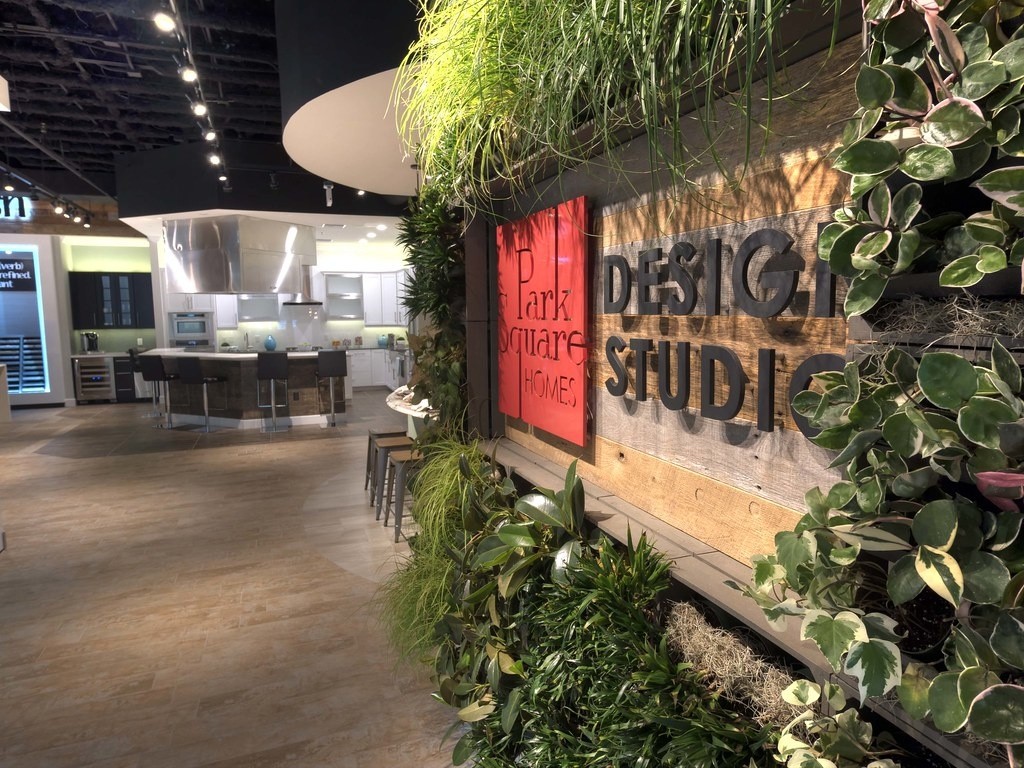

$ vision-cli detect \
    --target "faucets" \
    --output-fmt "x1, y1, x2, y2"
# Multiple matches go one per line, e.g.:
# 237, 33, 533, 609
243, 333, 254, 352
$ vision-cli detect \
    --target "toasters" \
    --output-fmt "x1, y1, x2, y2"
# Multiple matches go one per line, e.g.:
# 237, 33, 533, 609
81, 332, 105, 354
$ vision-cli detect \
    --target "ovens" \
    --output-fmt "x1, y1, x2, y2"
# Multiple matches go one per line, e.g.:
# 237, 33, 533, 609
72, 359, 115, 403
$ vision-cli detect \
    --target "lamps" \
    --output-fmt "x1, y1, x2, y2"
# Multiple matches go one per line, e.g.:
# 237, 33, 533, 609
153, 0, 231, 190
270, 172, 278, 189
4, 175, 16, 192
52, 195, 92, 229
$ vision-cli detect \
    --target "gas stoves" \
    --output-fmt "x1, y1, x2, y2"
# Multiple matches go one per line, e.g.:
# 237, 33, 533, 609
286, 346, 323, 351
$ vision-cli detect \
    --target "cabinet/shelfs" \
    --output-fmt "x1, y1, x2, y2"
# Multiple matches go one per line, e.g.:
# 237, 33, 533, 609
112, 356, 135, 402
347, 349, 387, 392
321, 270, 364, 320
132, 271, 155, 329
67, 271, 98, 331
214, 293, 238, 329
381, 268, 410, 327
168, 293, 214, 314
362, 272, 382, 327
385, 350, 400, 392
98, 272, 135, 330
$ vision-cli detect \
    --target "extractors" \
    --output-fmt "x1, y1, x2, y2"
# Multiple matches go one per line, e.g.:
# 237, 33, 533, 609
282, 293, 324, 305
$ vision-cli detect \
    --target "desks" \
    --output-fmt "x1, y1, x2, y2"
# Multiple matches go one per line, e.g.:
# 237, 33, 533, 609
386, 383, 440, 441
0, 364, 10, 416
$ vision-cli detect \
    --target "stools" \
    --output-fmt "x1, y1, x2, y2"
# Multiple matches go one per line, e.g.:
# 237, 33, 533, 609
315, 349, 348, 429
177, 357, 227, 433
139, 354, 187, 430
364, 427, 406, 501
370, 437, 416, 520
256, 351, 290, 433
384, 450, 425, 543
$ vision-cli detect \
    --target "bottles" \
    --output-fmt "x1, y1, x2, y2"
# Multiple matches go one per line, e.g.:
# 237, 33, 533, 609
378, 335, 387, 347
264, 336, 276, 350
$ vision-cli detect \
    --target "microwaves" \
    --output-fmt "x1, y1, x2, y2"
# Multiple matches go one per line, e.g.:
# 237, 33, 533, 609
168, 311, 217, 348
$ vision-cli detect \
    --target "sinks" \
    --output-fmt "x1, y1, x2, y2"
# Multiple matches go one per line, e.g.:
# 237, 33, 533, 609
228, 348, 257, 353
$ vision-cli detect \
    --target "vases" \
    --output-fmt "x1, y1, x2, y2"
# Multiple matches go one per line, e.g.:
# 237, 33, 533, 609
263, 336, 275, 351
